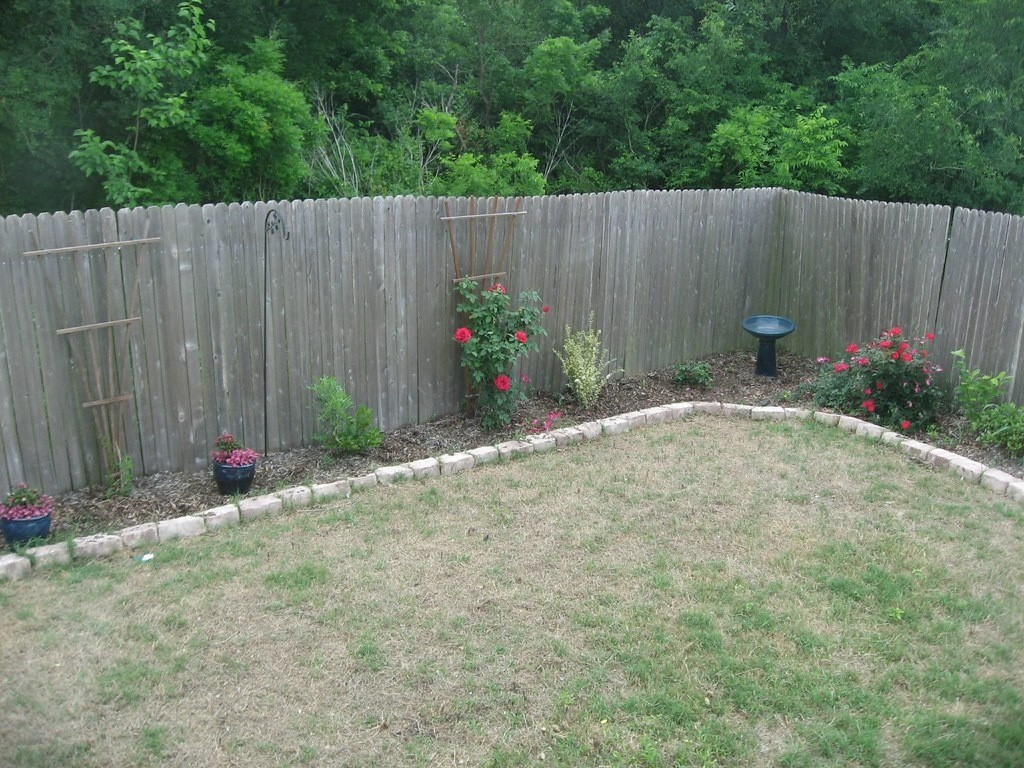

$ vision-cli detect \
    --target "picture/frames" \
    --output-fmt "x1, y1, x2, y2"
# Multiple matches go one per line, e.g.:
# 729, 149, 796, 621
212, 459, 255, 494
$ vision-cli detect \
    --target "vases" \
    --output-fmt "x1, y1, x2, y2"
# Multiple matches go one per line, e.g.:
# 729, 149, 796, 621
1, 512, 49, 542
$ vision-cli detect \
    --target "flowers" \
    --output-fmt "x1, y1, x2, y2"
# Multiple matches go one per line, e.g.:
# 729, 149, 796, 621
0, 480, 56, 520
211, 432, 258, 467
